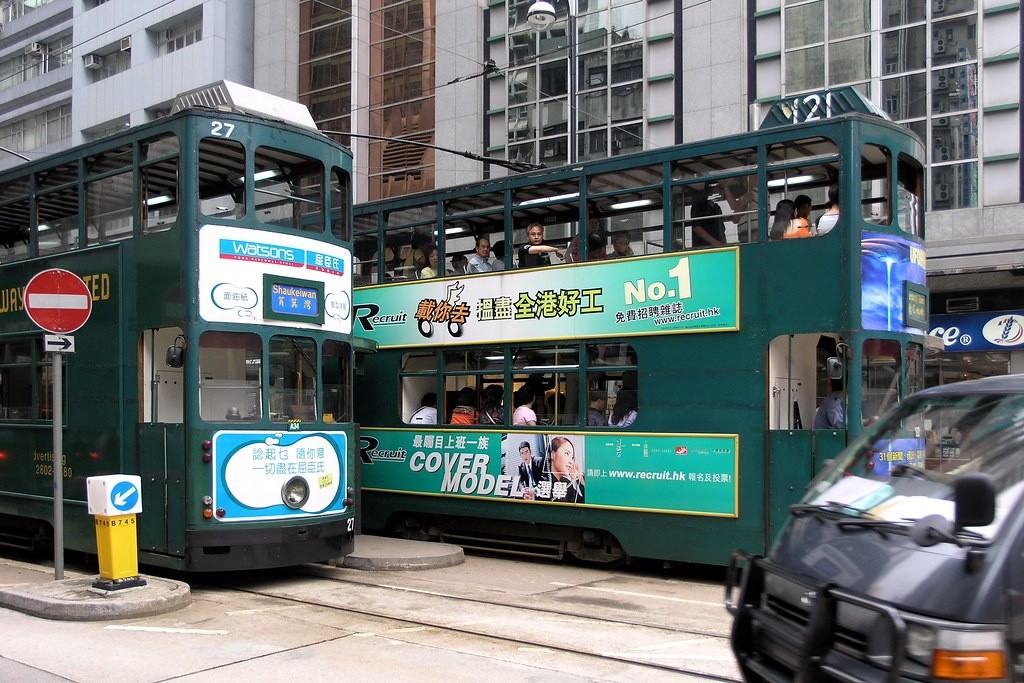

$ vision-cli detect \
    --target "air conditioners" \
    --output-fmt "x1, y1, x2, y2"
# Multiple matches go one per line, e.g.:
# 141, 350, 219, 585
934, 39, 946, 54
120, 37, 131, 54
84, 55, 104, 69
934, 110, 949, 127
933, 0, 945, 13
935, 75, 948, 91
935, 184, 950, 202
935, 147, 951, 163
24, 43, 47, 57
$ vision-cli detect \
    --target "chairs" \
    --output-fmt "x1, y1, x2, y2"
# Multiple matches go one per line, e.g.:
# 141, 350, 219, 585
393, 241, 575, 279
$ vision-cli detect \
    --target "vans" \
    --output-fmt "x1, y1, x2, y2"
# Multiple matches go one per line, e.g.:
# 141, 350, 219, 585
722, 372, 1024, 683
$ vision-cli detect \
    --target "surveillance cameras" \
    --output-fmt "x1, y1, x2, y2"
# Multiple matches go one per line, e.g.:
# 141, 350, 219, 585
527, 2, 557, 31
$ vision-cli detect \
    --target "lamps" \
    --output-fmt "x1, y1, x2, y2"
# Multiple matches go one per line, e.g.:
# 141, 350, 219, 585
28, 221, 63, 232
143, 190, 175, 206
227, 164, 292, 187
946, 297, 981, 313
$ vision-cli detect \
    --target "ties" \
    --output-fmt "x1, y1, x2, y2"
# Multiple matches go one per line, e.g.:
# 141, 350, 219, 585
528, 465, 535, 485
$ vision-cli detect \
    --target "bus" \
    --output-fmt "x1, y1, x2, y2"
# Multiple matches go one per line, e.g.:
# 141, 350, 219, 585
0, 79, 358, 573
264, 85, 945, 572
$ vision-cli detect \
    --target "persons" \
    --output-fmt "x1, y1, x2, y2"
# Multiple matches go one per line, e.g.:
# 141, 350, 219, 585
542, 436, 584, 502
812, 370, 875, 430
689, 179, 727, 248
401, 211, 634, 278
407, 347, 637, 426
517, 440, 545, 500
769, 179, 840, 240
718, 162, 772, 243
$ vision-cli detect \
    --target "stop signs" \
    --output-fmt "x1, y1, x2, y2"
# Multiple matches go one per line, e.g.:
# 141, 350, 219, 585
23, 267, 93, 336
